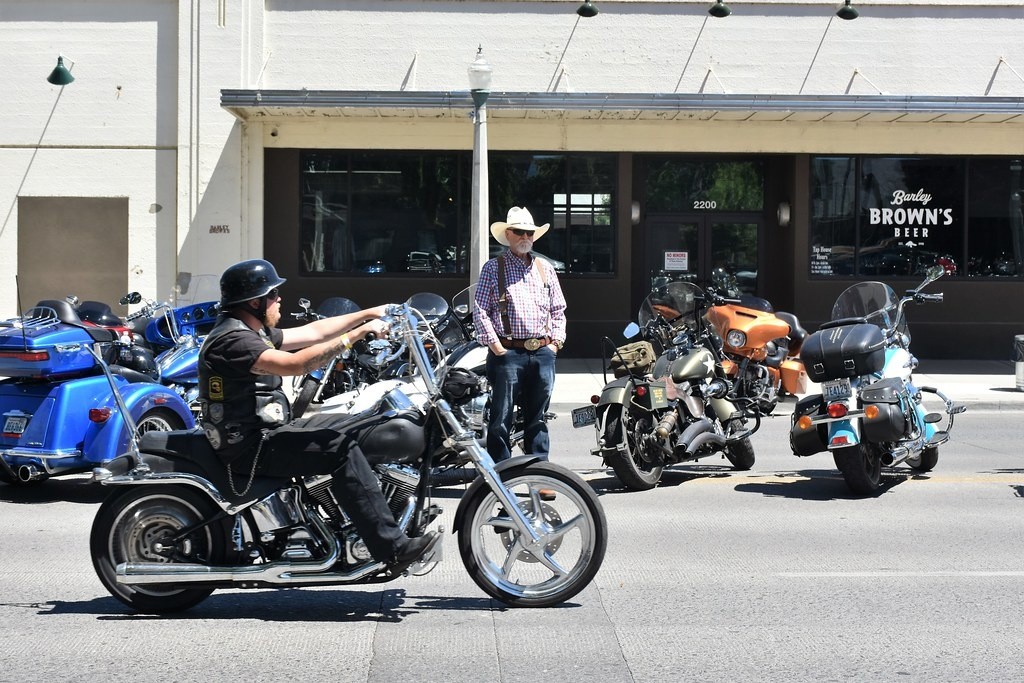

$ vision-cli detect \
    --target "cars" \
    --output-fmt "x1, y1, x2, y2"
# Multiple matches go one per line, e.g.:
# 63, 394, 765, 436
407, 244, 567, 275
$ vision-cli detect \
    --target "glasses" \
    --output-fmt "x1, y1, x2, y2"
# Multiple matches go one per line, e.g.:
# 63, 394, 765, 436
507, 228, 535, 237
267, 288, 278, 300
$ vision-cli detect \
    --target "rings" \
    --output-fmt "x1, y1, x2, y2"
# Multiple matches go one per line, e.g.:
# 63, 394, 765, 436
380, 326, 385, 331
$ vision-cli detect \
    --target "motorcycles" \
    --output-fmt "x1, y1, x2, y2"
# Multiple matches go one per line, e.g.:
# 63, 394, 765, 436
81, 298, 608, 616
650, 264, 809, 418
789, 260, 968, 496
0, 274, 557, 501
572, 278, 761, 492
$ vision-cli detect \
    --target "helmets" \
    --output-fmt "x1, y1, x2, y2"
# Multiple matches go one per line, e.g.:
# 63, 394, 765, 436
220, 260, 287, 307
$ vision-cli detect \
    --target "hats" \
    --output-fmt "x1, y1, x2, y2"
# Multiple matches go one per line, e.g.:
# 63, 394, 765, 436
490, 206, 550, 246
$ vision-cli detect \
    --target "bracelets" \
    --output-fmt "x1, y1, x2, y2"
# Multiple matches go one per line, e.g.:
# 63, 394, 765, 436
339, 333, 352, 350
551, 339, 563, 350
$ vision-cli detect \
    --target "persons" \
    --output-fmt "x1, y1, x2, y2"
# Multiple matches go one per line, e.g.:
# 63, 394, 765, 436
473, 207, 567, 501
198, 260, 439, 575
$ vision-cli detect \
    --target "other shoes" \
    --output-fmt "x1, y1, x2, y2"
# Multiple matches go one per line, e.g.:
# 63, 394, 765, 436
539, 489, 556, 500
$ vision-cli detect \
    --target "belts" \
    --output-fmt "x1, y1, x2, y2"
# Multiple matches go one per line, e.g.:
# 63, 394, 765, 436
499, 337, 550, 351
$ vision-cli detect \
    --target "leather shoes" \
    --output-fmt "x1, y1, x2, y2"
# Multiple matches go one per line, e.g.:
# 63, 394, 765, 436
406, 505, 438, 538
383, 531, 441, 577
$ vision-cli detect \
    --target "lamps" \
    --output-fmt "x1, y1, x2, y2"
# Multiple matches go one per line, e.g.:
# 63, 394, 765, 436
46, 54, 77, 87
839, 0, 858, 22
576, 1, 598, 17
707, 0, 730, 17
